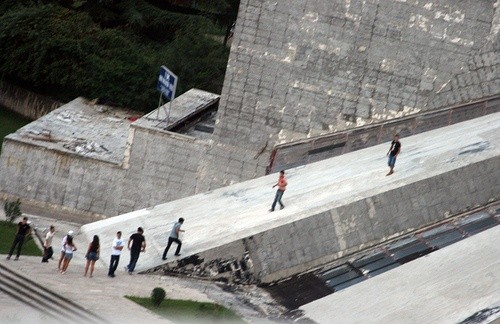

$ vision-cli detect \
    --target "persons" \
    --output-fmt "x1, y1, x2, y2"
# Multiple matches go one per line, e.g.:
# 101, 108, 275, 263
124, 226, 146, 275
386, 135, 401, 176
84, 235, 100, 278
57, 230, 77, 273
41, 227, 55, 263
162, 217, 185, 260
268, 170, 288, 212
108, 231, 124, 278
6, 217, 32, 261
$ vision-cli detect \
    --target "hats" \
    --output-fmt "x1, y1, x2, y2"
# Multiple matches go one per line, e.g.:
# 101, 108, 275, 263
67, 231, 74, 236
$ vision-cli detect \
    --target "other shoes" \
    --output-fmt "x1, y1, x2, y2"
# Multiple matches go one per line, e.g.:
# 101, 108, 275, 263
128, 271, 133, 275
173, 254, 180, 256
6, 256, 10, 260
269, 209, 274, 212
161, 258, 167, 260
14, 258, 18, 261
280, 207, 284, 210
62, 272, 65, 274
60, 270, 62, 273
57, 268, 60, 271
124, 266, 127, 271
